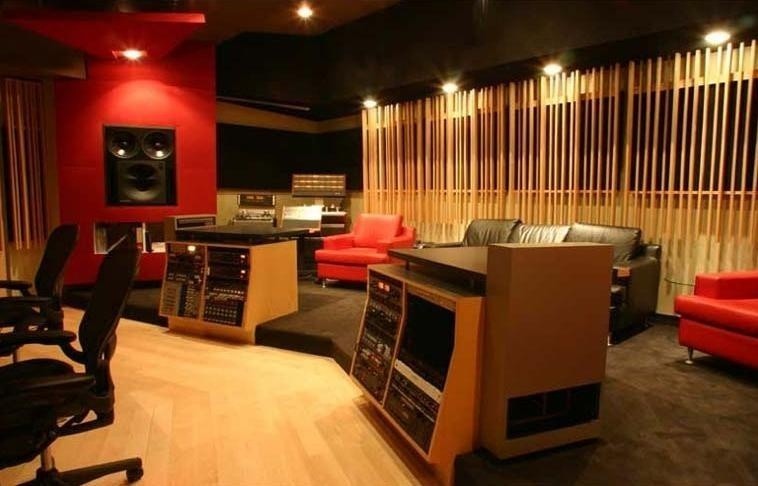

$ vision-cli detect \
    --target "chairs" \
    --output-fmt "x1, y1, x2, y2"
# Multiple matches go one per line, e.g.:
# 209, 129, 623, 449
0, 221, 86, 364
315, 211, 416, 288
0, 230, 147, 486
674, 270, 757, 374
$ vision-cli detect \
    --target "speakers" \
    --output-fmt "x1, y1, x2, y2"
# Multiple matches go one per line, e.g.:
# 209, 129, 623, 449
102, 123, 177, 207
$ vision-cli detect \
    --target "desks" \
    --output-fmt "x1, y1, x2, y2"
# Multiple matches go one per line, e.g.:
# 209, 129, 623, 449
185, 223, 311, 244
379, 233, 487, 289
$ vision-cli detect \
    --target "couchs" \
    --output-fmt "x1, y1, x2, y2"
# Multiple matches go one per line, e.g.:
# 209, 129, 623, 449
416, 214, 662, 347
